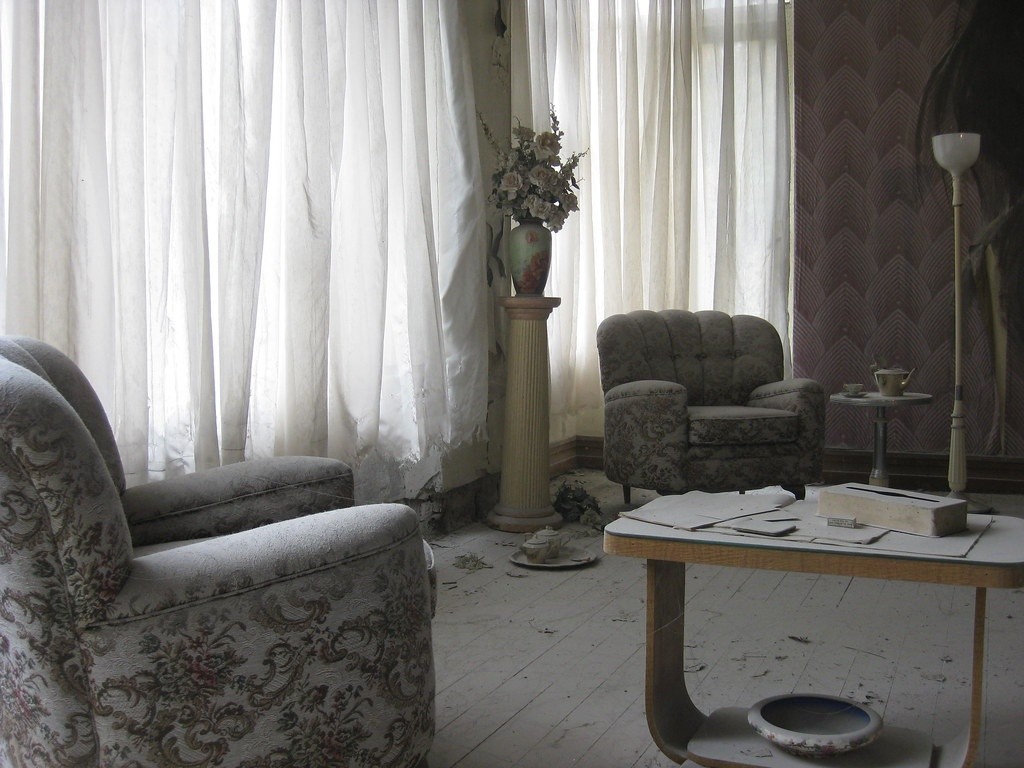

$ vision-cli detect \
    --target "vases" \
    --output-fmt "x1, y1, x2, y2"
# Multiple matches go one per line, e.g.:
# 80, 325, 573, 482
503, 214, 558, 299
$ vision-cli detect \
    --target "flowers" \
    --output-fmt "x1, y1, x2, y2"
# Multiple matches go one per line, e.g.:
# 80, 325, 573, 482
554, 478, 605, 534
482, 102, 589, 235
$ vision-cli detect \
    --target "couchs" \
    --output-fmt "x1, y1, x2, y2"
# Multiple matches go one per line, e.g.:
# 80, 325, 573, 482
594, 307, 826, 495
0, 334, 434, 768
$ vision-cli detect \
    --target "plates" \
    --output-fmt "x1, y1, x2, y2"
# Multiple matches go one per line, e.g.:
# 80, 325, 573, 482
839, 392, 868, 397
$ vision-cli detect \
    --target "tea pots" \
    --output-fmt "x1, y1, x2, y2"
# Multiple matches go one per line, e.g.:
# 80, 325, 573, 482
869, 364, 918, 397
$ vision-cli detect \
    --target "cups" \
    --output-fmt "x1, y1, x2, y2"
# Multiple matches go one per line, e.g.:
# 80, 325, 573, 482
843, 382, 864, 394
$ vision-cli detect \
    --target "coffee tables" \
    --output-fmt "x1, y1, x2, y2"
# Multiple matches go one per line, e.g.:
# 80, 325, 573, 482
830, 389, 933, 488
603, 490, 1024, 767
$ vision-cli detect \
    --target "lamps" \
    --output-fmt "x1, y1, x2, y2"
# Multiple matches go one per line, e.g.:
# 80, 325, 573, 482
930, 131, 994, 515
